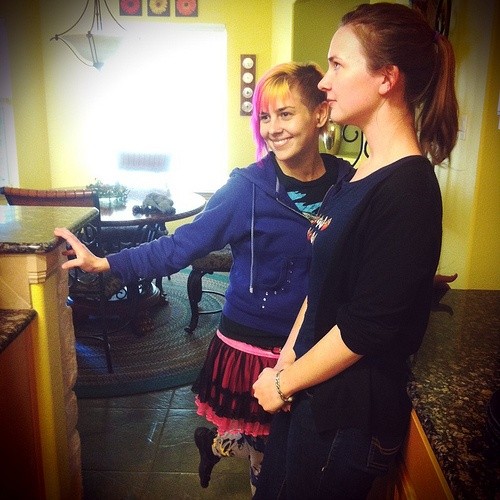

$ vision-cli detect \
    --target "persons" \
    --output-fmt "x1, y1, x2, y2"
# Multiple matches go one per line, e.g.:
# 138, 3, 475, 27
252, 2, 459, 500
54, 61, 357, 496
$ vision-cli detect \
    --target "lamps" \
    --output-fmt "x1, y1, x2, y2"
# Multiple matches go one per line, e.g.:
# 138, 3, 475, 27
49, 0, 126, 71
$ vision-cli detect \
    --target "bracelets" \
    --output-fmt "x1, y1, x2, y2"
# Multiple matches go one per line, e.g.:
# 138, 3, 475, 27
275, 369, 293, 402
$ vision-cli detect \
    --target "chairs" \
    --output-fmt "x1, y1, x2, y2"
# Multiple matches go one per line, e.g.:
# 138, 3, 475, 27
184, 243, 233, 332
0, 186, 146, 374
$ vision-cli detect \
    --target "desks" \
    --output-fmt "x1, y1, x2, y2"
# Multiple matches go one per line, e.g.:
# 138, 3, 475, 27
77, 191, 207, 337
